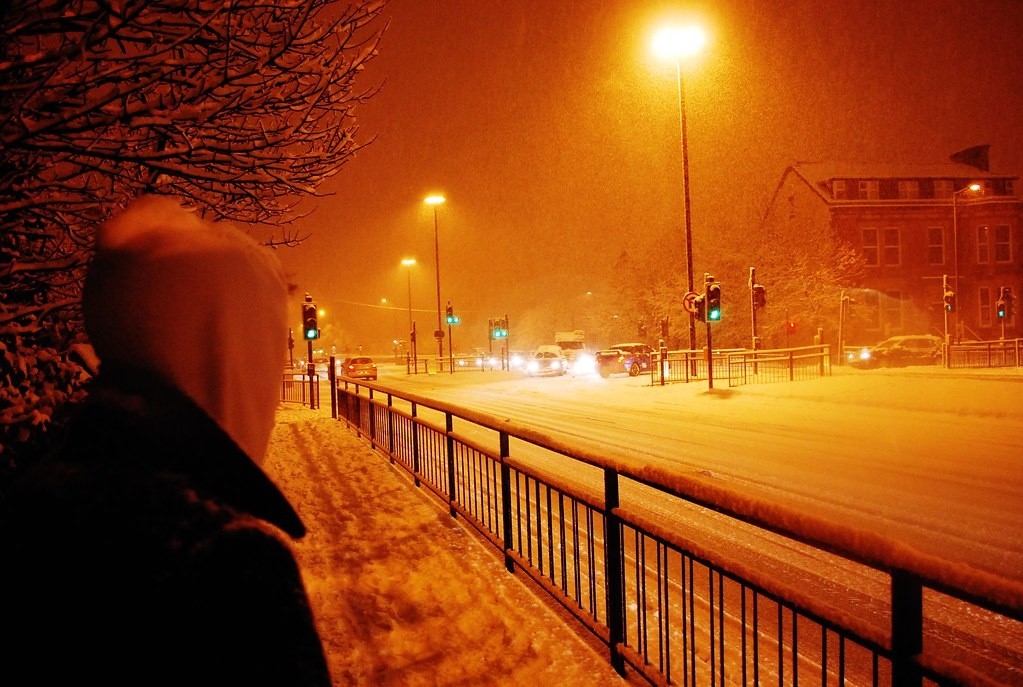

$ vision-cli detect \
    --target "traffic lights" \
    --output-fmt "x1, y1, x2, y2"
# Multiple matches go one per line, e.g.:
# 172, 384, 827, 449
494, 319, 507, 338
843, 295, 856, 327
996, 286, 1016, 320
302, 302, 318, 340
789, 321, 795, 333
753, 283, 766, 311
943, 289, 955, 312
446, 304, 460, 326
705, 282, 722, 322
288, 337, 294, 349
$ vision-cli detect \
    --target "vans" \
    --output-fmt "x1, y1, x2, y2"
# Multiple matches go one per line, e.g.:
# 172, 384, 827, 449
848, 334, 944, 370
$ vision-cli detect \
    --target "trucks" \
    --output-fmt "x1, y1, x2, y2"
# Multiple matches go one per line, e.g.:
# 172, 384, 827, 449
553, 331, 588, 372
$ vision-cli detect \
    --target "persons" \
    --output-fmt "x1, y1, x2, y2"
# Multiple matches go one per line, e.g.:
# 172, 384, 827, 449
0, 199, 332, 687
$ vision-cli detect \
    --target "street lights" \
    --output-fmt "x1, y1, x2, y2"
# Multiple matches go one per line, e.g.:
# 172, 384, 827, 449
318, 309, 325, 317
424, 195, 445, 371
400, 257, 414, 365
379, 297, 398, 362
654, 23, 698, 377
953, 183, 980, 340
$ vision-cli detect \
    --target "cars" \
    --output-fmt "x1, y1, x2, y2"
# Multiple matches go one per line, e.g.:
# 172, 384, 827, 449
340, 356, 377, 380
595, 343, 659, 378
505, 350, 526, 368
311, 358, 328, 372
469, 353, 496, 368
286, 359, 301, 368
453, 354, 469, 366
527, 346, 567, 377
